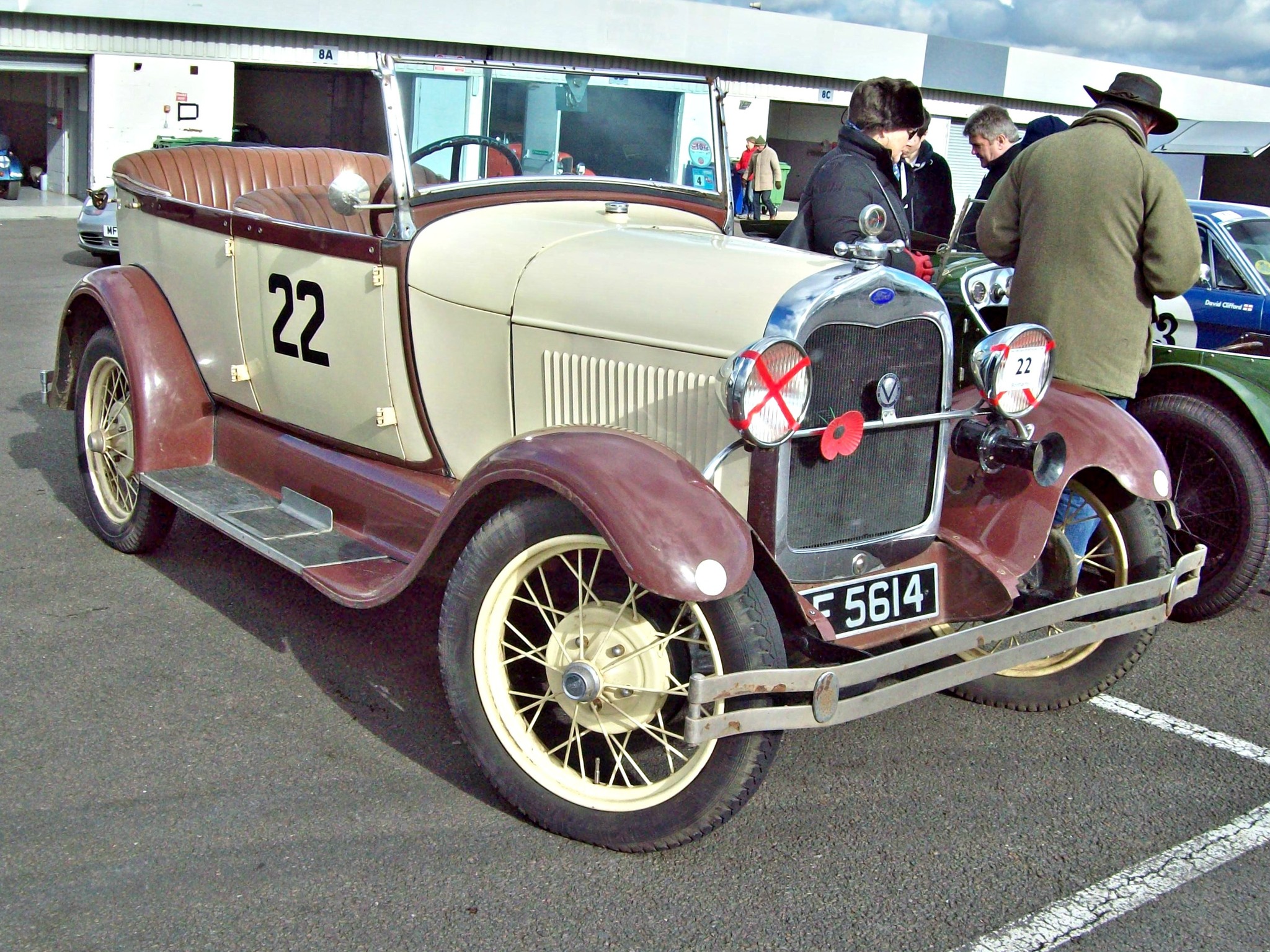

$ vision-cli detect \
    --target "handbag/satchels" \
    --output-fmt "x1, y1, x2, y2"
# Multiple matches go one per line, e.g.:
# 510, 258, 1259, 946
776, 199, 814, 250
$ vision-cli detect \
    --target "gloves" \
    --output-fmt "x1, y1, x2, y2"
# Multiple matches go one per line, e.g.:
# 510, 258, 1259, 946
904, 246, 935, 283
734, 164, 739, 170
741, 179, 746, 188
776, 181, 782, 189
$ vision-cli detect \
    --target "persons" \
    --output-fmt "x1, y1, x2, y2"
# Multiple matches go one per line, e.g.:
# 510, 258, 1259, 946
731, 136, 759, 221
741, 135, 784, 223
974, 68, 1205, 623
959, 104, 1020, 261
887, 106, 959, 257
785, 74, 939, 290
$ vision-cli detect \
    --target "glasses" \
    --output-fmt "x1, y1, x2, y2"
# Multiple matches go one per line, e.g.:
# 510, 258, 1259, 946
907, 130, 917, 138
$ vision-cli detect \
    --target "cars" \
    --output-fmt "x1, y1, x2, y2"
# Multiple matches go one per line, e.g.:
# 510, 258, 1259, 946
670, 197, 1270, 619
37, 35, 1205, 856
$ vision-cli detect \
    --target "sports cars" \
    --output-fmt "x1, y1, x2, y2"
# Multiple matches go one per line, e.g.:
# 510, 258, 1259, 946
75, 139, 281, 271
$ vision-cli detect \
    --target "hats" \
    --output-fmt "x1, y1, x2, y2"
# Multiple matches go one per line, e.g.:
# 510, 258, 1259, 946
746, 137, 757, 143
850, 76, 924, 129
755, 135, 766, 145
1084, 73, 1178, 135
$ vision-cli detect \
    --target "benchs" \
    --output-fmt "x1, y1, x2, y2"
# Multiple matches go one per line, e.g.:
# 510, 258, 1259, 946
112, 146, 441, 209
236, 185, 443, 243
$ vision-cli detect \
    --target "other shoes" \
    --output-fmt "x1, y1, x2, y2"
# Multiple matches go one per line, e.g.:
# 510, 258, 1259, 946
770, 207, 777, 220
737, 214, 754, 220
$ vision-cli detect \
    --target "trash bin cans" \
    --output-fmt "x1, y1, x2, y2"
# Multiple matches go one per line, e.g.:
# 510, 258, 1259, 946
728, 157, 791, 211
